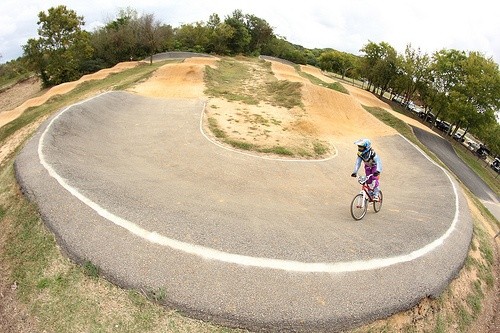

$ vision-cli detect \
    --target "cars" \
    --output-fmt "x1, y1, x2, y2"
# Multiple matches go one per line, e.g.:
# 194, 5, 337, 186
392, 94, 500, 173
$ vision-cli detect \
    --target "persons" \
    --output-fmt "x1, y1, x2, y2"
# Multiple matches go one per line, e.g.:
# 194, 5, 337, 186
351, 138, 382, 202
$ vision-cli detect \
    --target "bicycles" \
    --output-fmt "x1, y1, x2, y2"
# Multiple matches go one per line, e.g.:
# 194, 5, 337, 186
351, 173, 383, 220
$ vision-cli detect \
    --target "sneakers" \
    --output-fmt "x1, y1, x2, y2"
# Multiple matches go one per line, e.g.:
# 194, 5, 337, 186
372, 196, 379, 201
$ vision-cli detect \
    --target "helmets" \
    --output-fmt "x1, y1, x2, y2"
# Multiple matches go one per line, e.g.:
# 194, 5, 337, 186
354, 138, 371, 156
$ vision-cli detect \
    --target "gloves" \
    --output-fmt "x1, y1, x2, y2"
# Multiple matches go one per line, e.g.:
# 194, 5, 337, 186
373, 171, 379, 176
352, 172, 356, 177
